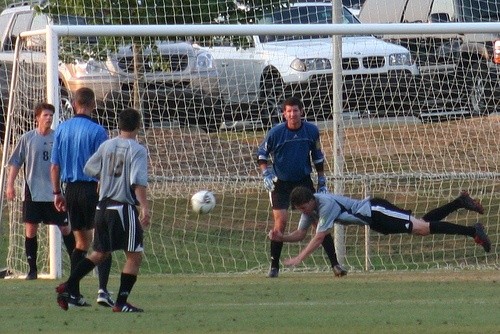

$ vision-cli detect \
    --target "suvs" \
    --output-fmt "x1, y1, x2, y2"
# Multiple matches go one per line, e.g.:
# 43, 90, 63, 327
0, 6, 219, 130
197, 7, 420, 124
357, 0, 499, 113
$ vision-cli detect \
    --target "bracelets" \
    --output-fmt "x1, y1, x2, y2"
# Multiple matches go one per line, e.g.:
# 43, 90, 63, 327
53, 189, 61, 195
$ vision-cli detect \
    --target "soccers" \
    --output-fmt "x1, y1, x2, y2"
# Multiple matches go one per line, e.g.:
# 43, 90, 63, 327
190, 190, 215, 214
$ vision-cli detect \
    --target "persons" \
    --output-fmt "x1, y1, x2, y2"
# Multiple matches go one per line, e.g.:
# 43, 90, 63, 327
54, 108, 149, 313
50, 88, 116, 306
257, 97, 347, 278
267, 186, 492, 267
5, 103, 79, 281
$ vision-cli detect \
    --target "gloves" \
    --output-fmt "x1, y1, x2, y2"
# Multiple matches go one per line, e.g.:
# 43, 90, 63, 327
317, 176, 328, 192
262, 170, 278, 192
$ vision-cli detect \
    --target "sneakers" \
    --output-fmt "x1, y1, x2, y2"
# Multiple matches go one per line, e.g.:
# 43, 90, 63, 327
472, 222, 491, 252
67, 292, 91, 307
269, 265, 280, 278
113, 301, 144, 312
55, 282, 69, 310
96, 289, 114, 307
26, 270, 38, 280
460, 190, 483, 215
332, 263, 347, 276
